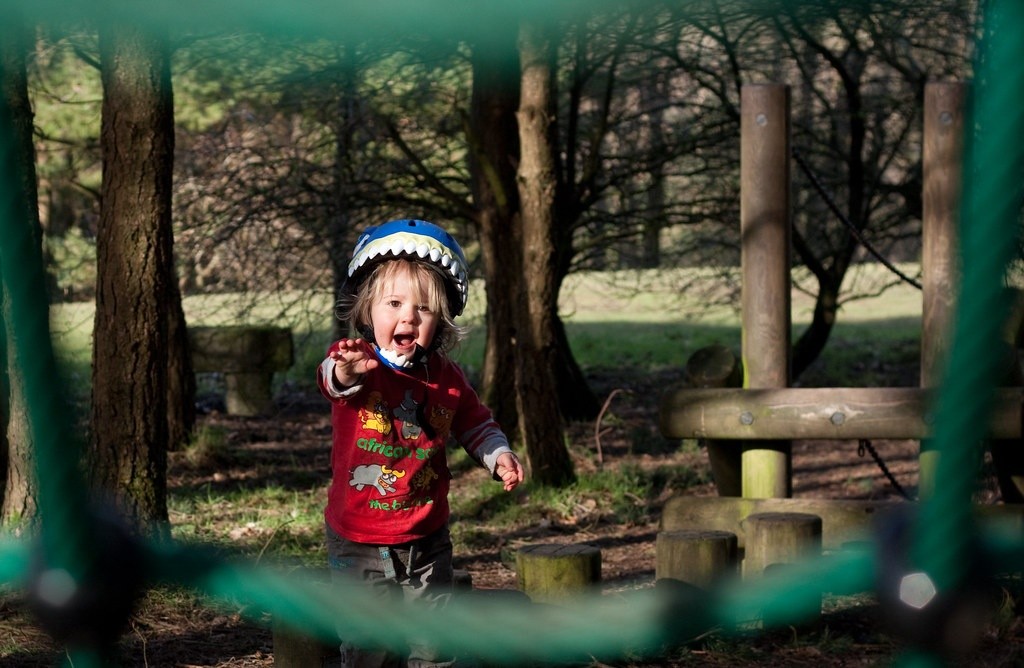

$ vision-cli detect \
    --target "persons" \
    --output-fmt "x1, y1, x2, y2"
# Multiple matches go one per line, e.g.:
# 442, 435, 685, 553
314, 216, 524, 668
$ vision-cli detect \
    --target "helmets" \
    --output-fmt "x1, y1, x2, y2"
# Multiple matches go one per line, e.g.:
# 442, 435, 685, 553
346, 218, 471, 373
